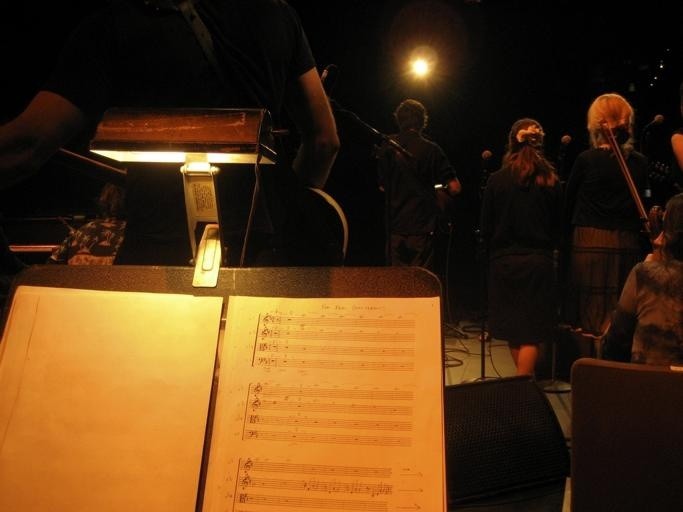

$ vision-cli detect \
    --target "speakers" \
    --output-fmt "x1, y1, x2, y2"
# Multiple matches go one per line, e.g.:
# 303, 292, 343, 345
445, 373, 570, 512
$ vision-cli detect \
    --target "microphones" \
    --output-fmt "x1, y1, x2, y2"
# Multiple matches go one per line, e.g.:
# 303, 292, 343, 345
557, 134, 573, 165
644, 113, 665, 134
482, 150, 493, 177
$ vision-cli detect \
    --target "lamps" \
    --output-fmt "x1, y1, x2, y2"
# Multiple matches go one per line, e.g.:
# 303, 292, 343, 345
89, 107, 276, 265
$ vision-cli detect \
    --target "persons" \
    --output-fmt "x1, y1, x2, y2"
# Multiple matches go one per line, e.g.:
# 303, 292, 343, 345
661, 82, 683, 195
45, 179, 132, 266
478, 116, 565, 380
563, 92, 650, 361
617, 189, 682, 368
374, 98, 463, 272
1, 0, 342, 266
